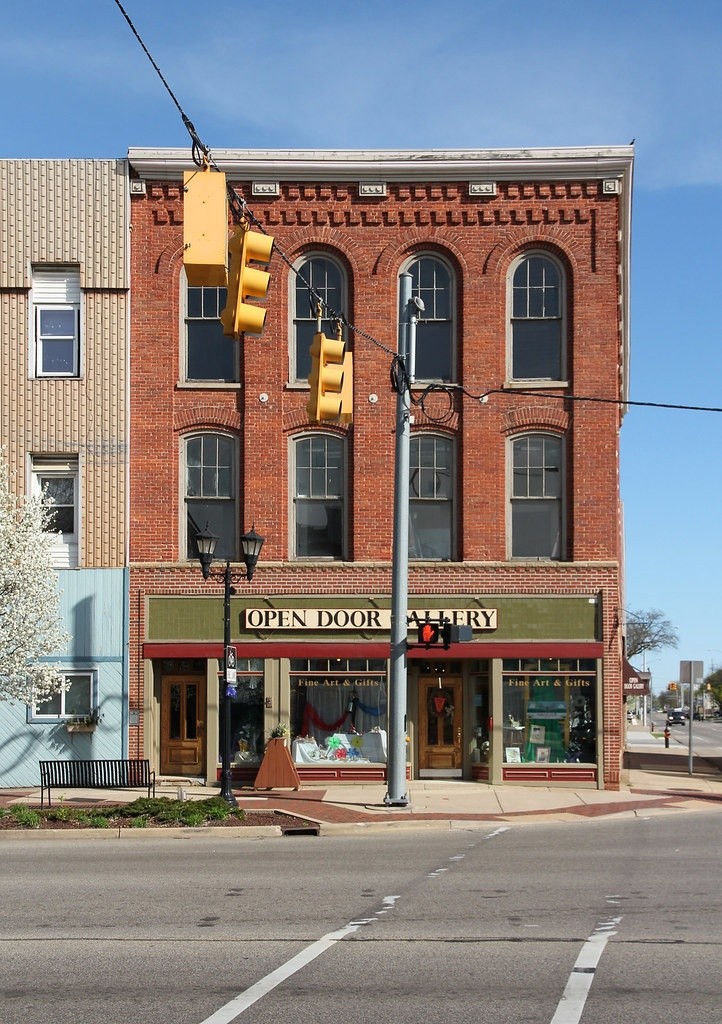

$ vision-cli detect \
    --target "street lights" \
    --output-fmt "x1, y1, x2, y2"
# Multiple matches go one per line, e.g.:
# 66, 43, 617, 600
197, 520, 266, 810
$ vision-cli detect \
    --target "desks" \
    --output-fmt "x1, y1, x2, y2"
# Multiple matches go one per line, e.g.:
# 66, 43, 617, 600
504, 727, 526, 760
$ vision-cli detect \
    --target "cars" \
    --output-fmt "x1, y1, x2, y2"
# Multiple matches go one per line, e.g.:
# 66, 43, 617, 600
667, 712, 685, 726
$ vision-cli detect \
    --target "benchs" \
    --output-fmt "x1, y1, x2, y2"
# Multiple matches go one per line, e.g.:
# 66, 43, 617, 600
39, 760, 156, 814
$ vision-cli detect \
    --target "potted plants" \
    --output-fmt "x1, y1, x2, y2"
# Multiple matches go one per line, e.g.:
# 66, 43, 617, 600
271, 725, 288, 747
58, 707, 104, 733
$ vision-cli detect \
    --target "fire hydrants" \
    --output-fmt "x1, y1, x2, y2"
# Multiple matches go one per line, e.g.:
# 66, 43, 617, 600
664, 726, 672, 748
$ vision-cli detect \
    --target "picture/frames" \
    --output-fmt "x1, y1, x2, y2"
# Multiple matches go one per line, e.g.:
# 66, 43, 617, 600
536, 747, 551, 763
530, 725, 545, 744
506, 748, 521, 763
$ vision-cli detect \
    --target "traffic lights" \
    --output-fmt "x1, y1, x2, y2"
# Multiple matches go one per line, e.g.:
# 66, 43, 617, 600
333, 321, 354, 427
418, 623, 439, 644
306, 301, 347, 426
669, 683, 676, 691
220, 213, 275, 339
